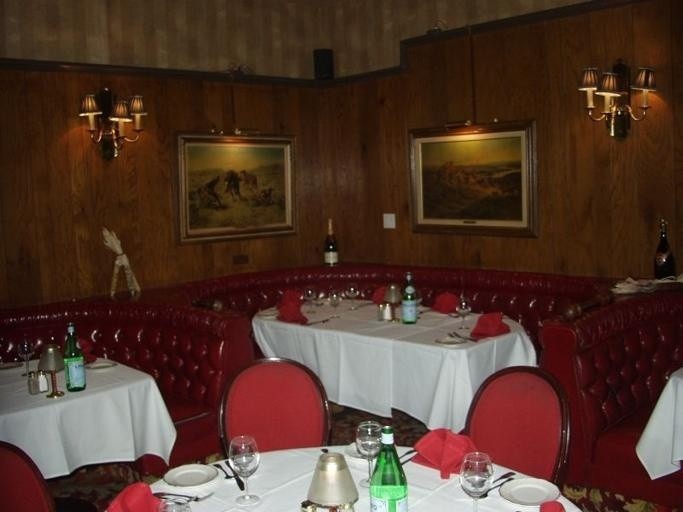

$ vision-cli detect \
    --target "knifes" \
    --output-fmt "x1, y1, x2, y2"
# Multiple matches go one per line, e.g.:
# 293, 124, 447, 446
223, 459, 246, 492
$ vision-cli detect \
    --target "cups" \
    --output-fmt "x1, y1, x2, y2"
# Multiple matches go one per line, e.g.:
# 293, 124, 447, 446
160, 499, 190, 510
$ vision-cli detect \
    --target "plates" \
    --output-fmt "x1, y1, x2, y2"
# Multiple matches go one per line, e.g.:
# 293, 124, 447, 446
85, 360, 117, 372
163, 463, 220, 488
434, 336, 466, 347
498, 476, 562, 508
346, 443, 377, 461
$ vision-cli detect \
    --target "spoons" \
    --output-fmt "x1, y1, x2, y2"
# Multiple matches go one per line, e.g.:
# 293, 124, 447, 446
478, 478, 513, 498
215, 464, 237, 480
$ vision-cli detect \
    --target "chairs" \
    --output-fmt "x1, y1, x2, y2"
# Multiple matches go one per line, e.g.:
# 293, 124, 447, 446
464, 367, 570, 485
217, 357, 332, 457
0, 440, 56, 512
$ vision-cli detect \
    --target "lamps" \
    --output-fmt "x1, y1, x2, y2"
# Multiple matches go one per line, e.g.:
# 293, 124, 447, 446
384, 283, 401, 323
579, 60, 656, 139
79, 87, 147, 157
37, 344, 65, 398
308, 452, 358, 512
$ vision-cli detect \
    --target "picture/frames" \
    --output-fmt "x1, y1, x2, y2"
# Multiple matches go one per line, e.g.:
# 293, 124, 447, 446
176, 129, 297, 243
407, 120, 538, 238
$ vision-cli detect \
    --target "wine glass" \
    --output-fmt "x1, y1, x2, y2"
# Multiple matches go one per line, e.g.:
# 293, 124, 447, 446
357, 420, 380, 490
259, 284, 360, 327
17, 342, 35, 376
460, 453, 494, 512
457, 296, 472, 330
229, 436, 262, 506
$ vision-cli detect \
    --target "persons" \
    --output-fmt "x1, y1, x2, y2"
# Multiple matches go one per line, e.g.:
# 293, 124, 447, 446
197, 168, 259, 209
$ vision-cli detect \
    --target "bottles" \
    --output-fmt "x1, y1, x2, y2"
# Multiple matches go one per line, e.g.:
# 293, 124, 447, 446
63, 323, 86, 392
369, 425, 409, 510
27, 371, 38, 394
377, 271, 417, 324
192, 299, 226, 312
36, 371, 50, 393
323, 218, 338, 267
654, 220, 675, 278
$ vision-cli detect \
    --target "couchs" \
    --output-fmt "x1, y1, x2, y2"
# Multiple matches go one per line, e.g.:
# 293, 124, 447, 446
538, 292, 682, 506
0, 296, 252, 478
166, 264, 624, 353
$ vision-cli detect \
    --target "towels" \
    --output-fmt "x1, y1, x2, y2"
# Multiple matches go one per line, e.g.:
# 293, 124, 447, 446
372, 288, 385, 303
430, 291, 459, 314
470, 311, 510, 338
105, 482, 166, 512
277, 288, 307, 323
411, 429, 476, 478
